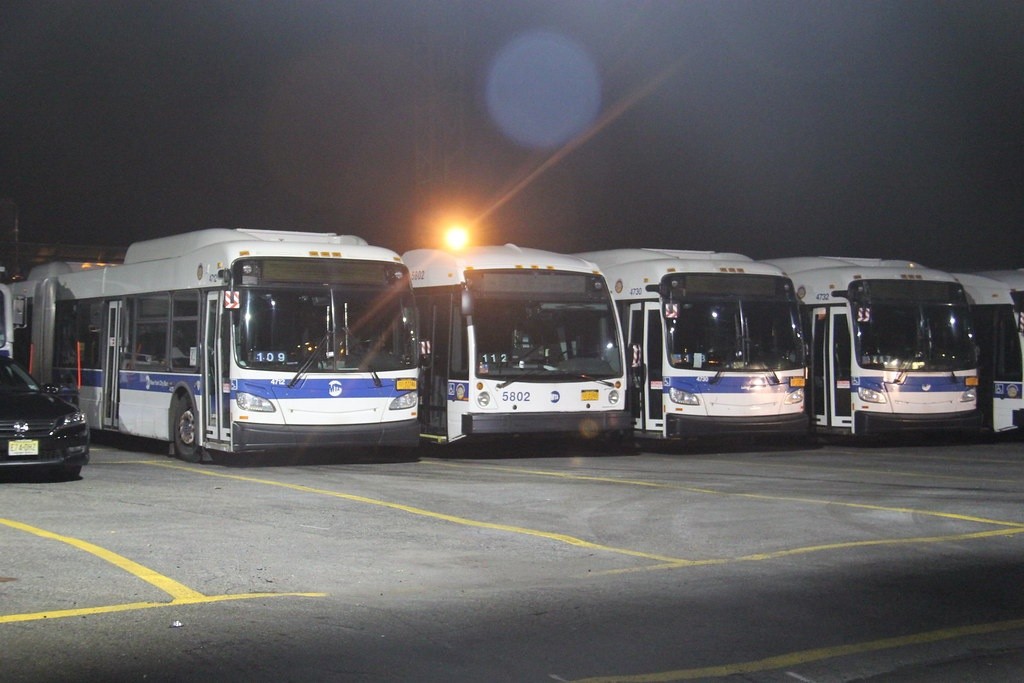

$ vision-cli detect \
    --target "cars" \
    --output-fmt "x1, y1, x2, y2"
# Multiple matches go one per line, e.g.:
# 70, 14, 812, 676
0, 354, 92, 484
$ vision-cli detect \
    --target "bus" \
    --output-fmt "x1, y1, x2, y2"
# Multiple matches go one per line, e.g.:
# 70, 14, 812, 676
945, 269, 1024, 439
566, 247, 812, 445
749, 256, 984, 441
399, 241, 642, 456
9, 226, 423, 463
